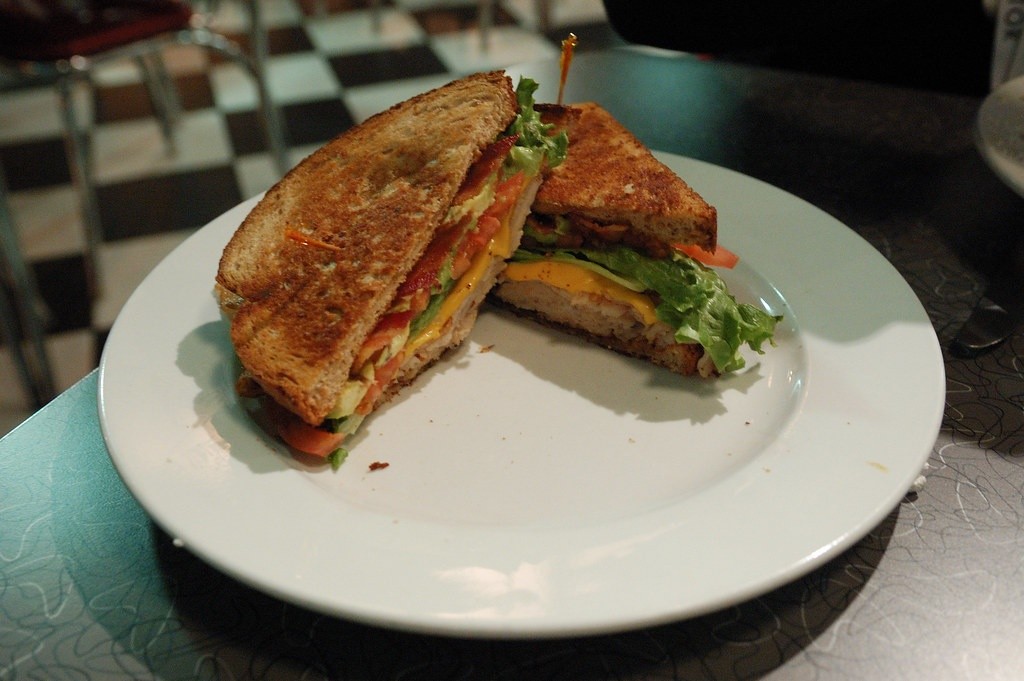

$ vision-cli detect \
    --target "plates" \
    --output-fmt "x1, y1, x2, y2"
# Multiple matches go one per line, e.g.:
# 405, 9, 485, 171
974, 77, 1024, 197
98, 150, 946, 638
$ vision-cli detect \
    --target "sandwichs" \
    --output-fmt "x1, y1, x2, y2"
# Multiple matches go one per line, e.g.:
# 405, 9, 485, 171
211, 68, 571, 459
486, 102, 783, 380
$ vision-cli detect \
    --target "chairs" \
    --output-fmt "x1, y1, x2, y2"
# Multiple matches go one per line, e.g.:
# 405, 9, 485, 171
0, 0, 289, 303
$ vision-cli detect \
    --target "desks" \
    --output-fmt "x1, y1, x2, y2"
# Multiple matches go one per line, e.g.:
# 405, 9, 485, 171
0, 48, 1023, 681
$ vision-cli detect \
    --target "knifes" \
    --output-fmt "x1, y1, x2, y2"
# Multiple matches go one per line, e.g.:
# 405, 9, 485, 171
954, 229, 1019, 347
